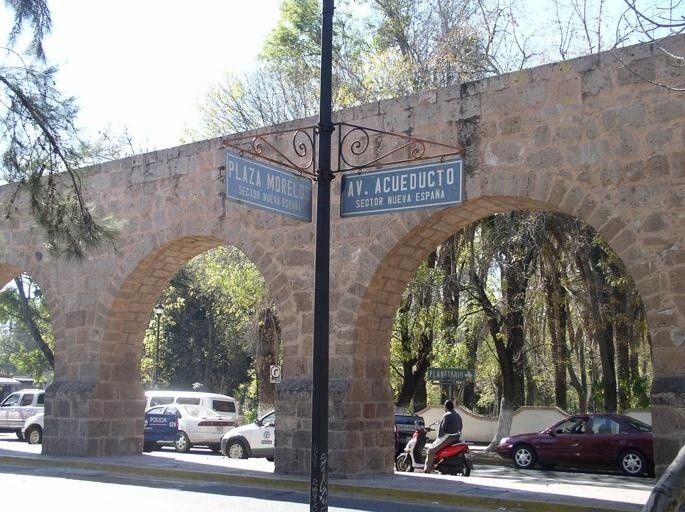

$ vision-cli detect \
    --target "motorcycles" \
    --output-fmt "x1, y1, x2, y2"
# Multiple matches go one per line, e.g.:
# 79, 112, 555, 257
397, 414, 475, 476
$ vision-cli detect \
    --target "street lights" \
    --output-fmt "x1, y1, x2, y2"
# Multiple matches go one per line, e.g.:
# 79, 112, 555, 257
151, 300, 166, 390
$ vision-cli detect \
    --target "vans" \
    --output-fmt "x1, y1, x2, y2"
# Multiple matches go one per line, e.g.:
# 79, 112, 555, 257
143, 391, 239, 430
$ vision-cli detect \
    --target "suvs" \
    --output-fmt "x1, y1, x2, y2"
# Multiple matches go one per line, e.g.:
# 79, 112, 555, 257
0, 388, 46, 439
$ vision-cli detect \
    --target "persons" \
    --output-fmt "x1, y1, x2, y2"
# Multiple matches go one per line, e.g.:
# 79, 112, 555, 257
420, 399, 463, 473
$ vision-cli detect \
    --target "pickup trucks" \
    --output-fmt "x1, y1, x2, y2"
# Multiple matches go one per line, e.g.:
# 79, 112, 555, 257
392, 411, 427, 463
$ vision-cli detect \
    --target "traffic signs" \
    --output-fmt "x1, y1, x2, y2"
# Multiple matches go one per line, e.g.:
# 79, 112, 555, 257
427, 366, 477, 383
335, 157, 466, 218
222, 151, 314, 224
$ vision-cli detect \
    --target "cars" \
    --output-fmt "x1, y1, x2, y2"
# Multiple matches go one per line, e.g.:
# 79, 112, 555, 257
221, 407, 276, 463
641, 442, 685, 512
492, 411, 656, 477
142, 404, 235, 454
21, 412, 45, 445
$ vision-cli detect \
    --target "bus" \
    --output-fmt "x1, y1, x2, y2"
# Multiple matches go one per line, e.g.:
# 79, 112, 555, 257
0, 376, 37, 412
